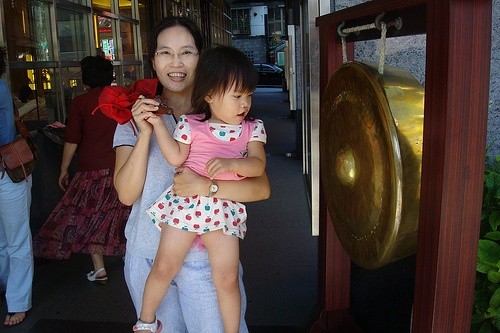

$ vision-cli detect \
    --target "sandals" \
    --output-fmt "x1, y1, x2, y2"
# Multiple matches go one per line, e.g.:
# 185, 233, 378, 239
87, 268, 108, 284
133, 312, 163, 333
3, 312, 26, 327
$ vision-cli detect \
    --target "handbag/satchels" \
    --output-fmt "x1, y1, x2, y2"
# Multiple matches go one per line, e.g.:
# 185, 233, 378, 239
0, 133, 40, 183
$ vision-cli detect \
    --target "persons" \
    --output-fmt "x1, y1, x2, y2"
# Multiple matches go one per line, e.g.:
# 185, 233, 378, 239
32, 55, 133, 282
132, 45, 267, 333
0, 44, 33, 327
113, 17, 271, 333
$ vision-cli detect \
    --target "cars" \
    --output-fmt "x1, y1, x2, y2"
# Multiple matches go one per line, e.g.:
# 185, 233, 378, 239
253, 63, 284, 86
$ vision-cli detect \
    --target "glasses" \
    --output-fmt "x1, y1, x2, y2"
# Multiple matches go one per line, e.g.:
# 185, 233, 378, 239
155, 49, 197, 59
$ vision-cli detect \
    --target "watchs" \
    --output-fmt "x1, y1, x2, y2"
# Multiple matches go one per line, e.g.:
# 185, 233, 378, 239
209, 181, 218, 197
